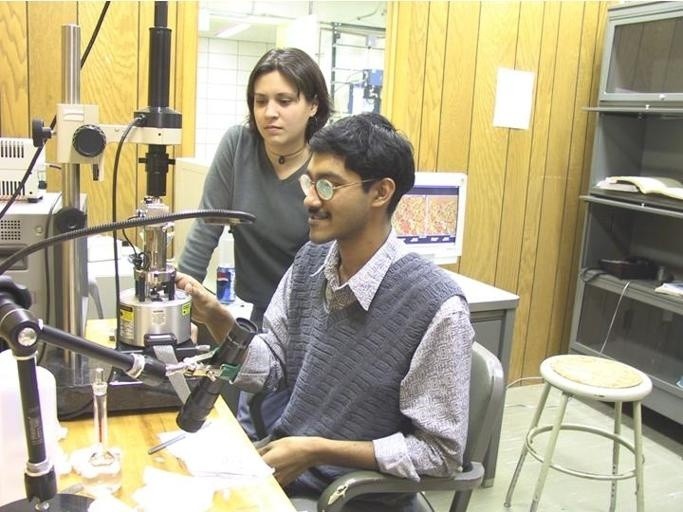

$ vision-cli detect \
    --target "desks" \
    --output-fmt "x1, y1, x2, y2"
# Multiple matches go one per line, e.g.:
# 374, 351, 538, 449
35, 312, 303, 510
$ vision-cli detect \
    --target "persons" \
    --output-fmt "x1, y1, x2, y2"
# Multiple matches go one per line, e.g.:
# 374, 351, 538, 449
176, 48, 337, 436
174, 113, 475, 510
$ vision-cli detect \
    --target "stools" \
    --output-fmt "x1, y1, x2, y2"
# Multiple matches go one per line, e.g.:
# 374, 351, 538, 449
499, 355, 653, 512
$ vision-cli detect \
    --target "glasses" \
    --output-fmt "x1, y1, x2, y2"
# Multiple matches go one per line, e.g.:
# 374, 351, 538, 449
296, 174, 378, 201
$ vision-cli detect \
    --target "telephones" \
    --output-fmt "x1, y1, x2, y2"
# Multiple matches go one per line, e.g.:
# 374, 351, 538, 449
599, 256, 658, 279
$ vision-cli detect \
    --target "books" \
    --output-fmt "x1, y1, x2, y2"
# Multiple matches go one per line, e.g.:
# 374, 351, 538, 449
596, 175, 683, 200
653, 281, 683, 299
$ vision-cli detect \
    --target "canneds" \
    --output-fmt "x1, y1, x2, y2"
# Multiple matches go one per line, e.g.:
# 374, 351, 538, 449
217, 267, 235, 305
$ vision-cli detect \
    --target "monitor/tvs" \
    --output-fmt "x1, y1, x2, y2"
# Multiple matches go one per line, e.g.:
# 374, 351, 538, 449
391, 172, 467, 264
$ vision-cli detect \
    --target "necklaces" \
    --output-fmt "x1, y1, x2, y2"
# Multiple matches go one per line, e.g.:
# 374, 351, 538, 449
268, 143, 307, 165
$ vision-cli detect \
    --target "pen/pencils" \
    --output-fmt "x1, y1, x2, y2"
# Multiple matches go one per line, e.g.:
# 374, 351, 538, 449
148, 434, 186, 454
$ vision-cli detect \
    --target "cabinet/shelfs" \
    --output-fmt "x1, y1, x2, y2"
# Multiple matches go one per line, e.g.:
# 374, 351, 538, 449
568, 1, 683, 428
438, 264, 517, 490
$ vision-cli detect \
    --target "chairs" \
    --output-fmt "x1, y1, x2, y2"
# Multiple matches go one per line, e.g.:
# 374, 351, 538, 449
306, 340, 503, 512
